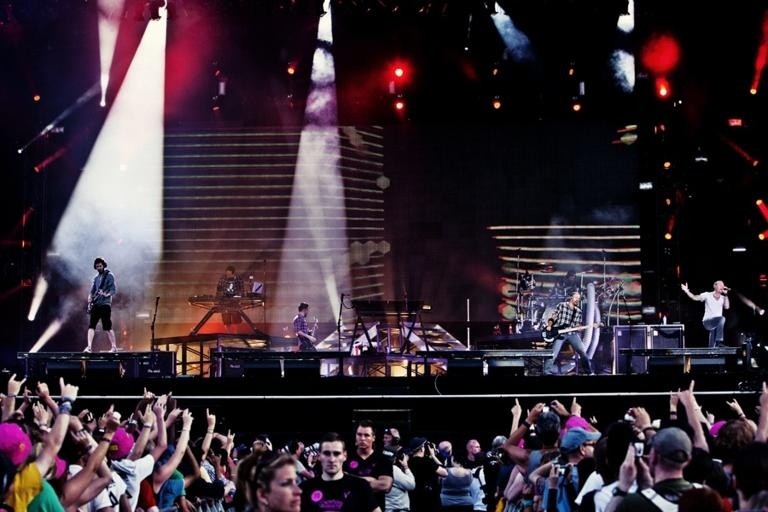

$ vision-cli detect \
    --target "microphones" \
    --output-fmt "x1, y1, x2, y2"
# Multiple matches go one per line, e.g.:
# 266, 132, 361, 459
621, 295, 627, 302
603, 250, 607, 255
723, 288, 731, 290
156, 297, 160, 308
341, 293, 344, 302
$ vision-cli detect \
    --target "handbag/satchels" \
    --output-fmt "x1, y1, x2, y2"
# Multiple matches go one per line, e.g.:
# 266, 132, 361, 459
503, 465, 527, 501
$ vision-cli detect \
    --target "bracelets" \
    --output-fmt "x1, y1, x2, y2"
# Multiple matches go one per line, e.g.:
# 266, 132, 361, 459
685, 289, 689, 292
723, 294, 728, 296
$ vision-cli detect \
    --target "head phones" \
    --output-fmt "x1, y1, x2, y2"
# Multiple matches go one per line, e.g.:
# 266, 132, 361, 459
392, 437, 400, 445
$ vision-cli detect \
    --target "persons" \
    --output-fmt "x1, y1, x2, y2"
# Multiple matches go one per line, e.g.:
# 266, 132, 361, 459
293, 302, 319, 352
542, 291, 597, 377
217, 266, 244, 335
84, 258, 117, 354
3, 371, 767, 510
552, 269, 579, 297
681, 280, 730, 357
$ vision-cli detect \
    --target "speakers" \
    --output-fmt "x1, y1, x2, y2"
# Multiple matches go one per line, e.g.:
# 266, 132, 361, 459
488, 360, 524, 377
86, 360, 123, 378
284, 360, 320, 378
243, 360, 281, 378
46, 360, 85, 376
447, 359, 484, 378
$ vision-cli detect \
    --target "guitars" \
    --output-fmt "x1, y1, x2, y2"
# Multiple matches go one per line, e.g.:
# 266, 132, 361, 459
542, 323, 604, 343
299, 316, 319, 349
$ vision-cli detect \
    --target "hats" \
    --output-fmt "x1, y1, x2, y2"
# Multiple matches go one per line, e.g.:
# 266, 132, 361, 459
382, 428, 426, 459
1, 422, 31, 466
108, 428, 135, 458
708, 420, 727, 437
648, 427, 691, 466
533, 410, 602, 450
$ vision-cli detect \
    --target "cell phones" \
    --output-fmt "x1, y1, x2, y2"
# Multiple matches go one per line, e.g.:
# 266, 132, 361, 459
625, 413, 636, 421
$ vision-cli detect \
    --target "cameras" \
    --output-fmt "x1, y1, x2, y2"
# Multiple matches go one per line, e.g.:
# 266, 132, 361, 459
555, 466, 567, 476
424, 442, 439, 452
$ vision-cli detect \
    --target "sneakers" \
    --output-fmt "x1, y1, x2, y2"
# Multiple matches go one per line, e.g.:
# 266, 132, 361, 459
112, 347, 117, 352
83, 346, 92, 353
